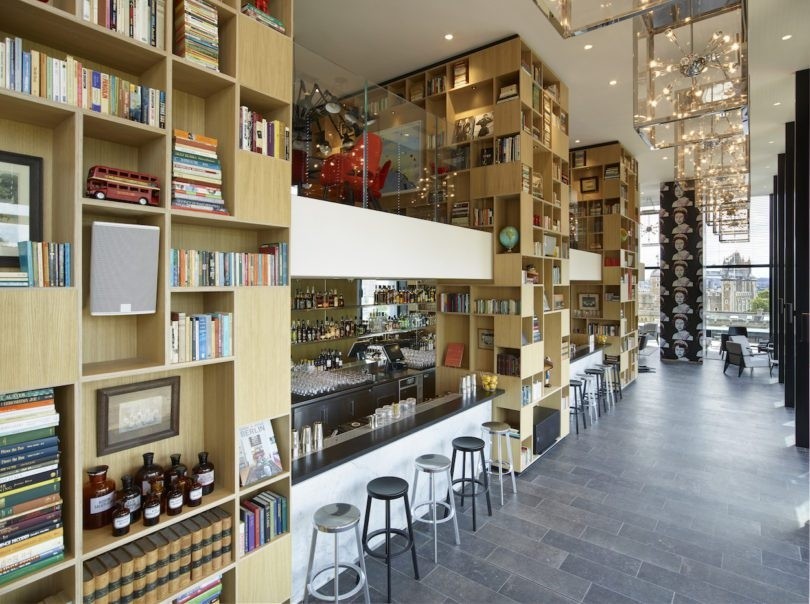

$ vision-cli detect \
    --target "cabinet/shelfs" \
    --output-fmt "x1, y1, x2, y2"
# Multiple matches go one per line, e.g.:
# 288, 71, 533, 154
0, 0, 295, 604
568, 141, 640, 389
293, 278, 439, 439
290, 385, 505, 604
298, 32, 574, 473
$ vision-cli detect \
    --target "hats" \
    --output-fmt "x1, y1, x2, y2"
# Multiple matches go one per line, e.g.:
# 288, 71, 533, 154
674, 287, 688, 294
674, 207, 688, 215
673, 261, 688, 268
673, 340, 688, 348
672, 314, 688, 321
673, 234, 688, 241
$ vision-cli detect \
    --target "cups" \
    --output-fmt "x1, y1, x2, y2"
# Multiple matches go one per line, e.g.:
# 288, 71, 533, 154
291, 371, 372, 395
301, 424, 312, 454
313, 422, 324, 452
458, 373, 476, 389
370, 398, 416, 429
292, 429, 300, 459
400, 347, 435, 370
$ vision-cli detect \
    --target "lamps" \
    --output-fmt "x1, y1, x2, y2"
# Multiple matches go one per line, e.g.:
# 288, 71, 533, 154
297, 78, 378, 156
534, 0, 751, 243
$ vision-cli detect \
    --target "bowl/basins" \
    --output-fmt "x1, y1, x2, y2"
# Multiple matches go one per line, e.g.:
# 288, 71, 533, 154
481, 372, 500, 392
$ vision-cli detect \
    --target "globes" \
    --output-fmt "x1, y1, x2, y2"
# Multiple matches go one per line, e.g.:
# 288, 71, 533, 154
500, 226, 519, 253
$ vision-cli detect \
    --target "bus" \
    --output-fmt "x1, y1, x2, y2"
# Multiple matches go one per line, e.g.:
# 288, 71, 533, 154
87, 165, 161, 206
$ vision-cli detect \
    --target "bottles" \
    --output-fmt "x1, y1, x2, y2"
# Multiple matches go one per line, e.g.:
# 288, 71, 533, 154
419, 334, 433, 350
187, 475, 203, 506
142, 490, 161, 526
165, 483, 183, 516
171, 467, 192, 505
146, 481, 166, 514
374, 284, 436, 304
115, 475, 142, 526
136, 453, 165, 503
84, 465, 116, 530
165, 453, 187, 493
291, 309, 435, 344
191, 452, 215, 495
294, 286, 345, 309
111, 497, 130, 537
291, 348, 344, 372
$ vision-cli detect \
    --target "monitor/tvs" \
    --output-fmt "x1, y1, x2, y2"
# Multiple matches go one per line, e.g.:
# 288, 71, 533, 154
382, 343, 406, 373
346, 340, 370, 361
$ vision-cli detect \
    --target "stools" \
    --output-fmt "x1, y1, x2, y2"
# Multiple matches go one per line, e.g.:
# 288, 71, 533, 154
444, 437, 492, 532
303, 503, 370, 604
473, 422, 518, 506
569, 360, 623, 436
356, 476, 420, 604
409, 454, 462, 564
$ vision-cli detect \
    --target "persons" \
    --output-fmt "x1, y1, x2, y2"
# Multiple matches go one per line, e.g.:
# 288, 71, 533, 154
476, 114, 493, 137
455, 119, 471, 142
661, 180, 704, 362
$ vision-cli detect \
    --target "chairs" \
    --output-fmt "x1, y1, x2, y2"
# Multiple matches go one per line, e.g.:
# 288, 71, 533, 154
638, 323, 660, 349
720, 324, 779, 378
291, 131, 392, 210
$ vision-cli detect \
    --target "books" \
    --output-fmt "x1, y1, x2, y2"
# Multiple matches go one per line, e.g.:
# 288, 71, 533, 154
357, 97, 387, 114
561, 269, 638, 367
241, 3, 285, 33
495, 134, 521, 164
497, 353, 520, 376
427, 130, 444, 148
522, 385, 532, 405
452, 202, 494, 225
439, 292, 516, 315
0, 240, 71, 287
42, 0, 218, 74
240, 490, 287, 557
522, 162, 544, 200
0, 36, 165, 130
239, 106, 290, 160
533, 315, 541, 342
36, 490, 235, 603
0, 389, 65, 583
409, 60, 469, 102
172, 128, 289, 366
522, 61, 566, 147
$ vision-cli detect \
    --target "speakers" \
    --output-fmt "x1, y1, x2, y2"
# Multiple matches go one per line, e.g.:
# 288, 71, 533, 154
90, 221, 160, 316
